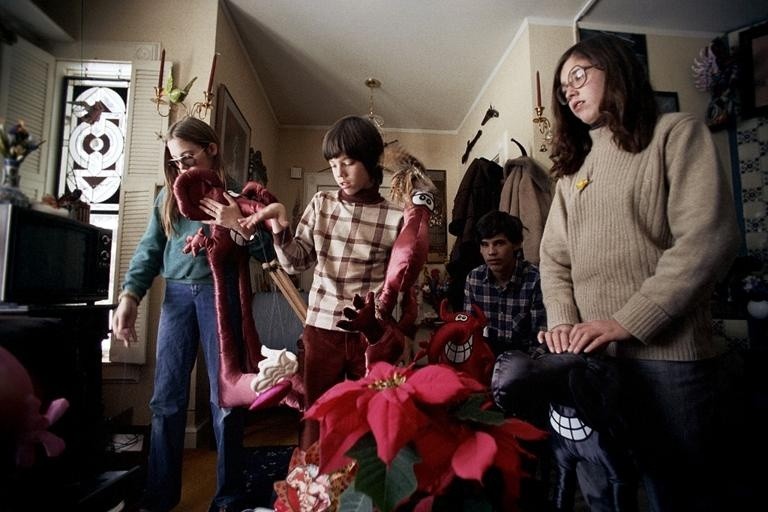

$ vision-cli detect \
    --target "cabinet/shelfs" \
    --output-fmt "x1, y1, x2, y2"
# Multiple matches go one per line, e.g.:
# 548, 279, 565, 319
0, 303, 130, 449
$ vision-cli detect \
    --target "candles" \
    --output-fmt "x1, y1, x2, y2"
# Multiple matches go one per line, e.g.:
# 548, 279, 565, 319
155, 48, 218, 101
532, 69, 552, 108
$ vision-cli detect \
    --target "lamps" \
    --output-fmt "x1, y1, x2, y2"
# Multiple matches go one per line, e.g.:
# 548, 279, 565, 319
364, 77, 385, 142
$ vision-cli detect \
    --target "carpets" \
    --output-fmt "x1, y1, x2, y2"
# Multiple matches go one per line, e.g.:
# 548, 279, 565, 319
207, 443, 296, 512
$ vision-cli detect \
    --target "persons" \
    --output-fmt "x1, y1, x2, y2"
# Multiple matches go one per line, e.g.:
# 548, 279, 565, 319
105, 117, 258, 511
538, 34, 747, 511
462, 210, 548, 349
238, 114, 413, 457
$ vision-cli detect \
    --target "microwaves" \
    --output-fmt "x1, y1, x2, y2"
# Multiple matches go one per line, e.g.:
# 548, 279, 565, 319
0, 199, 114, 312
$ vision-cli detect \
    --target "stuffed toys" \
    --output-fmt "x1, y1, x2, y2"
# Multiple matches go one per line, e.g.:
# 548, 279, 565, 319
173, 161, 304, 413
335, 151, 440, 363
487, 330, 667, 511
421, 300, 490, 377
1, 348, 68, 472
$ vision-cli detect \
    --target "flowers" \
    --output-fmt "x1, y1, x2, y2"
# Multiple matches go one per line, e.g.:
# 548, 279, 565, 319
273, 360, 555, 512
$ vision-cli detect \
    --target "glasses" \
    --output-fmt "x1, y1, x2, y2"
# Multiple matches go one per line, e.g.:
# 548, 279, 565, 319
555, 64, 601, 105
169, 149, 204, 169
